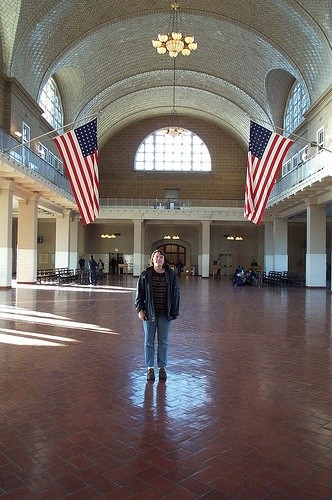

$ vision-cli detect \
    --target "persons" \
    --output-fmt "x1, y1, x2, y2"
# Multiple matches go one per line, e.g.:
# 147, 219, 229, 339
232, 259, 258, 286
79, 255, 124, 285
164, 259, 184, 278
134, 250, 180, 380
216, 259, 221, 279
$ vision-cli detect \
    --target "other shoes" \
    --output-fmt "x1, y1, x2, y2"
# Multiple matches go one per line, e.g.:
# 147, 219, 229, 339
159, 367, 167, 379
147, 368, 155, 379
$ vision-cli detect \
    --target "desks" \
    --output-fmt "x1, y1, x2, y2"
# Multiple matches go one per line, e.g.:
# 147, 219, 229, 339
116, 263, 129, 281
209, 264, 224, 283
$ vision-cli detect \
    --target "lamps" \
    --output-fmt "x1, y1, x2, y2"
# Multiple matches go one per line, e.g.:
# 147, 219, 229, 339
148, 0, 201, 139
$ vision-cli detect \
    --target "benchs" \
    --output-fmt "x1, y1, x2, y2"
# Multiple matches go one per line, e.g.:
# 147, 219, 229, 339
36, 266, 104, 286
262, 270, 307, 288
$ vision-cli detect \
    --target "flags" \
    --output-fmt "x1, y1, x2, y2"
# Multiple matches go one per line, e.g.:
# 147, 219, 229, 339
52, 116, 100, 225
243, 119, 295, 226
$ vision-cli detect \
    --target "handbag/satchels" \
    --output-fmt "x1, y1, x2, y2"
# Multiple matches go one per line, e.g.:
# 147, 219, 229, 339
99, 262, 102, 269
